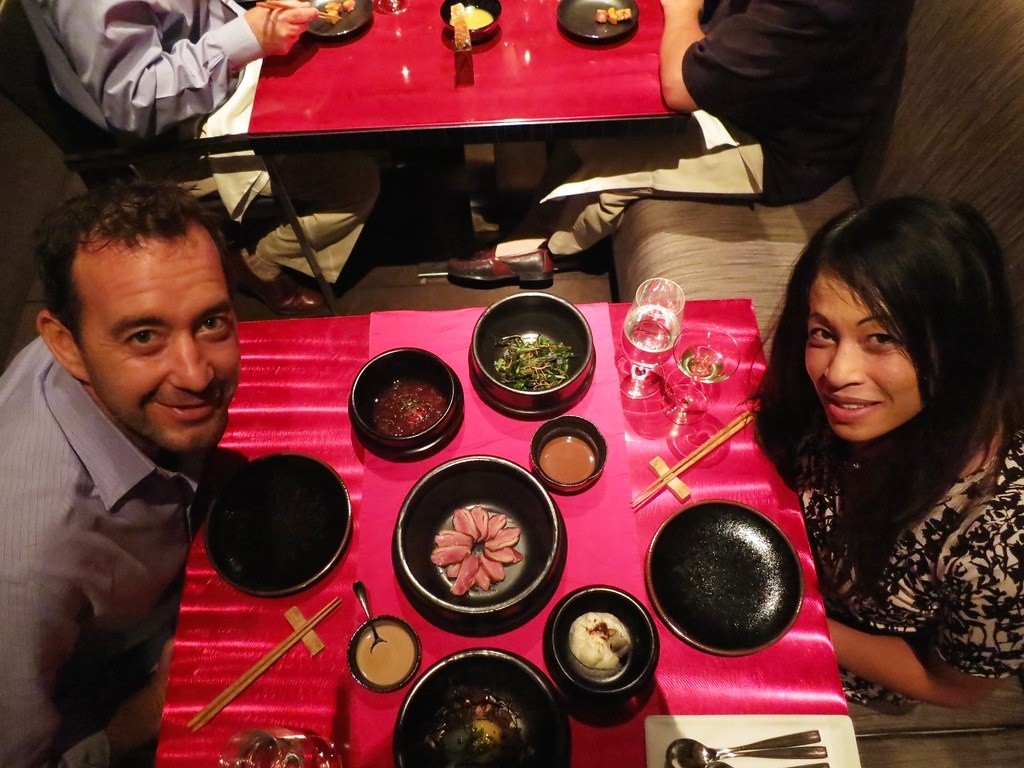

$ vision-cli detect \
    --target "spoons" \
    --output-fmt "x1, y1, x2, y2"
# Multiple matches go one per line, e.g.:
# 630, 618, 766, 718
665, 731, 830, 768
353, 582, 387, 654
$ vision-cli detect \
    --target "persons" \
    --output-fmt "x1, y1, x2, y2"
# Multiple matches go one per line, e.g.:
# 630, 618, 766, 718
20, 0, 381, 313
0, 188, 241, 768
447, 0, 913, 281
754, 196, 1024, 713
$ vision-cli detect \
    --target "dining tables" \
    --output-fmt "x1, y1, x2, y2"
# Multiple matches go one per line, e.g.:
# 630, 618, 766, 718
157, 297, 862, 768
248, 0, 691, 267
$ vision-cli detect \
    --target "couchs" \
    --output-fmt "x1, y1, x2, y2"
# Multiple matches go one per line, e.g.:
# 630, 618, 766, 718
610, 0, 1024, 768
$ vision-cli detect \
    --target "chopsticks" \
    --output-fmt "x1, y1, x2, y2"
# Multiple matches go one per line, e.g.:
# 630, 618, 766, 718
188, 597, 342, 733
256, 2, 341, 21
631, 412, 753, 511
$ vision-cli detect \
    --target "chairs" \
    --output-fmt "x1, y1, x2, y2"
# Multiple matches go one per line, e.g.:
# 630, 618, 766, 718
0, 0, 342, 316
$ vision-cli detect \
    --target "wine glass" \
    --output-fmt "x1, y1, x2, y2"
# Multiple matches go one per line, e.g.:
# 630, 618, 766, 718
663, 326, 741, 425
619, 278, 685, 398
376, 0, 410, 14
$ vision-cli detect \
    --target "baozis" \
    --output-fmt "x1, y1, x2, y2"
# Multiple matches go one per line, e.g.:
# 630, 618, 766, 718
568, 610, 631, 671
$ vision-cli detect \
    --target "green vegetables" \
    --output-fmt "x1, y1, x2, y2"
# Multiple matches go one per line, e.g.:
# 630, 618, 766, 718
493, 334, 574, 390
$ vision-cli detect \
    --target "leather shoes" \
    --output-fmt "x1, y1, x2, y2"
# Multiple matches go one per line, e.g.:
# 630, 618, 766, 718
239, 260, 325, 314
447, 243, 554, 285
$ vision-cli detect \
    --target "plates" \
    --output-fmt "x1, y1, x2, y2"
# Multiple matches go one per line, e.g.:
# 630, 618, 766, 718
645, 715, 862, 768
647, 499, 805, 657
296, 0, 373, 38
204, 453, 352, 594
558, 0, 639, 39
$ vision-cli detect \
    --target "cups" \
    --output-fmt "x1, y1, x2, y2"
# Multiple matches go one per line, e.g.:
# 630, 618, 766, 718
219, 727, 346, 768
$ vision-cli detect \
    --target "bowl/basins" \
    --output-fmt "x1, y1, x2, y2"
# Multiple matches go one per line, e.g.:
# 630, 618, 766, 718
543, 585, 659, 701
529, 415, 608, 494
468, 293, 598, 420
389, 649, 572, 768
438, 0, 504, 43
347, 346, 463, 461
392, 454, 567, 638
347, 616, 421, 693
